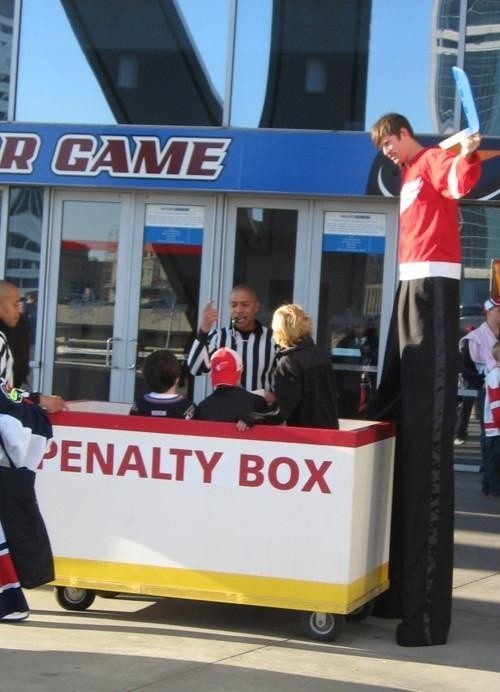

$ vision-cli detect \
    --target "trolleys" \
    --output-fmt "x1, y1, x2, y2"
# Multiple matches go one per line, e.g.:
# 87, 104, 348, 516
14, 400, 397, 641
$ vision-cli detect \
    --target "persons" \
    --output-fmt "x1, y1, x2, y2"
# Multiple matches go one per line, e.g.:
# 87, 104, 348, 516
126, 348, 199, 421
458, 296, 500, 434
0, 372, 55, 623
364, 110, 483, 647
185, 284, 283, 409
454, 324, 484, 447
236, 302, 341, 433
484, 342, 500, 497
0, 278, 72, 414
334, 313, 378, 420
192, 346, 271, 426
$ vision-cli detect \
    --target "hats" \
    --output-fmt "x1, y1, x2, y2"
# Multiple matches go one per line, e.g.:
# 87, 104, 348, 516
210, 347, 243, 386
